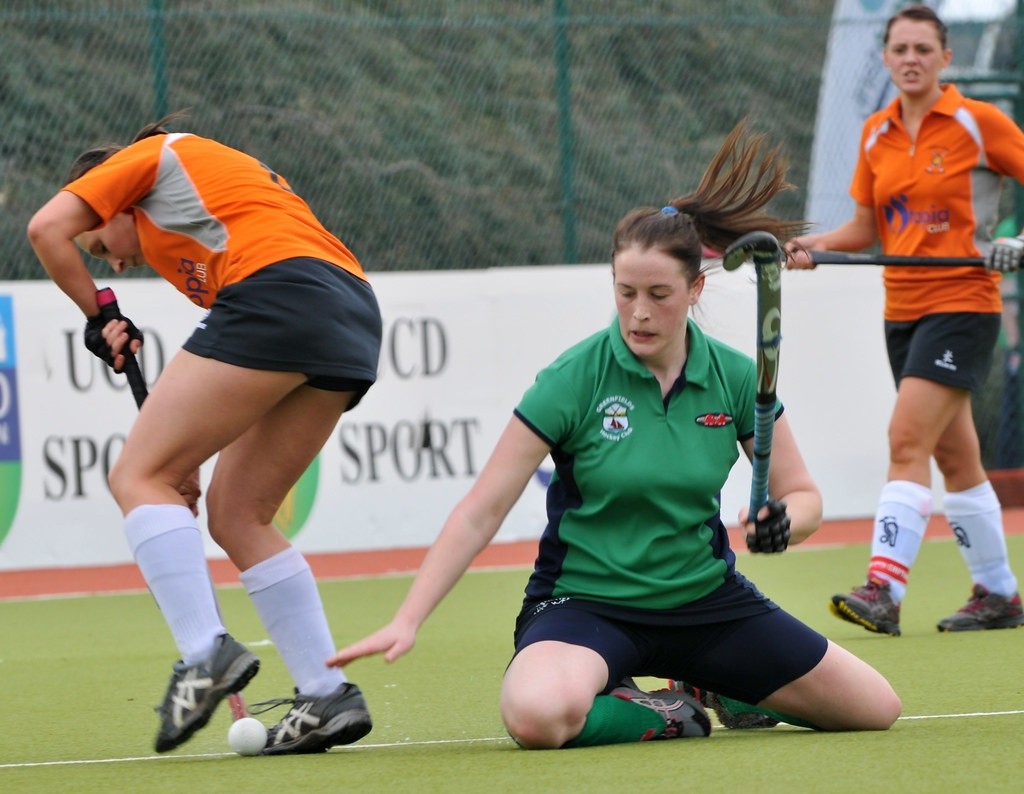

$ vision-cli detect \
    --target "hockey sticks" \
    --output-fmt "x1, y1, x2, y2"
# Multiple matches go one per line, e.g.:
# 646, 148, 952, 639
722, 230, 783, 524
94, 286, 247, 721
808, 249, 1024, 271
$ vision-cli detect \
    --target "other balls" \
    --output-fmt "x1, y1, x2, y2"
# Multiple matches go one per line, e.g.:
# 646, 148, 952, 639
229, 717, 266, 755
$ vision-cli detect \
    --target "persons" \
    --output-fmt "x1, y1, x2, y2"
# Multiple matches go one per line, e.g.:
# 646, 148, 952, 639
325, 114, 904, 751
782, 4, 1024, 637
26, 109, 383, 754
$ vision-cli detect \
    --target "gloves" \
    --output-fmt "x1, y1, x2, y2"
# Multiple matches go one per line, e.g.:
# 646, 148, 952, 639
984, 235, 1024, 273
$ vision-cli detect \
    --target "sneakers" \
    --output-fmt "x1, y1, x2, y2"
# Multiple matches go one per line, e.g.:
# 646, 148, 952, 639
669, 679, 780, 729
154, 629, 261, 753
829, 573, 901, 638
247, 682, 372, 756
604, 673, 711, 741
937, 583, 1024, 632
